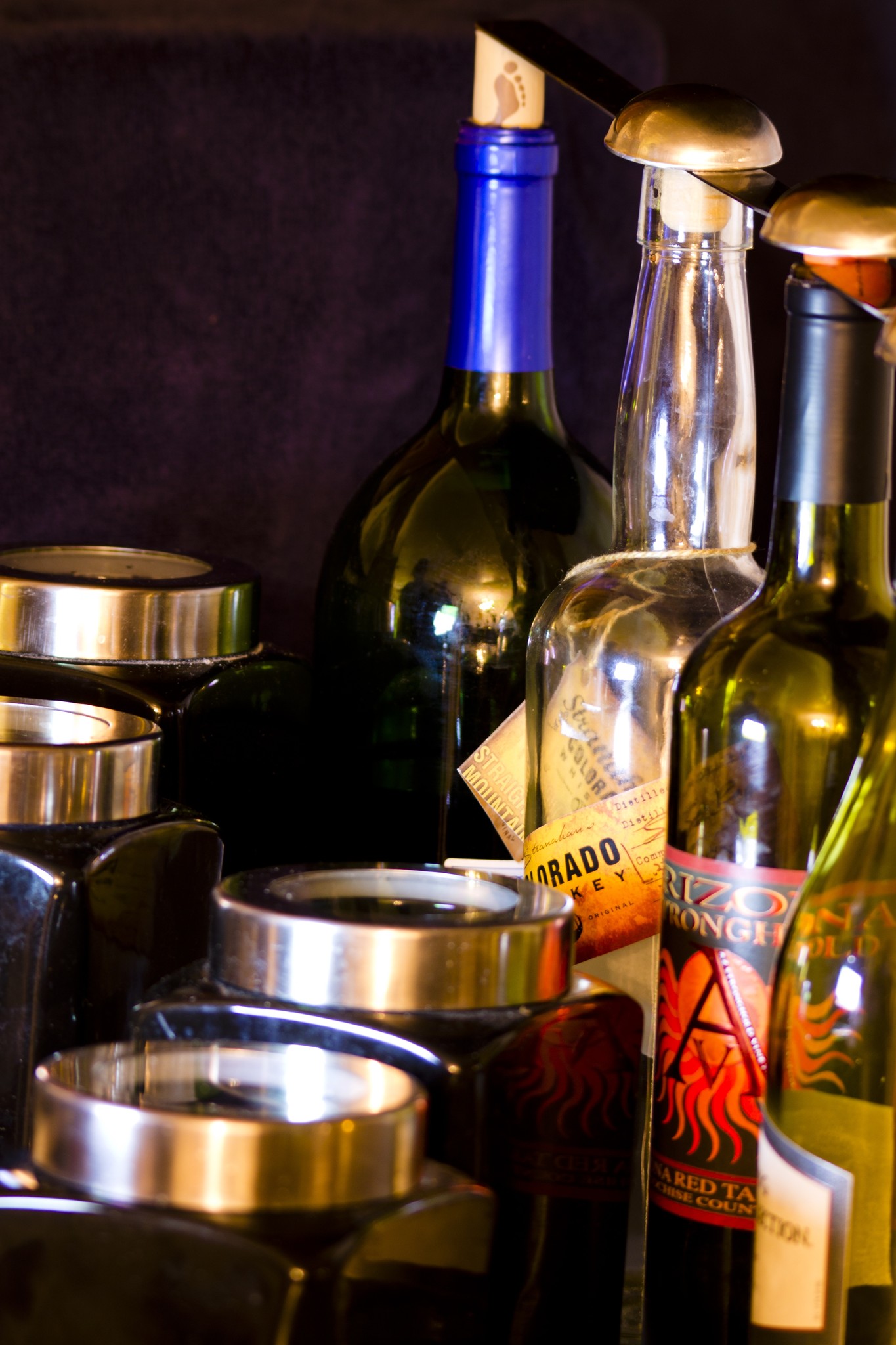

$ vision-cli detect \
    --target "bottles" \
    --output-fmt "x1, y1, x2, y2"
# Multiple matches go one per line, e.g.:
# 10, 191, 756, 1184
493, 109, 780, 1006
303, 131, 615, 879
646, 262, 895, 1337
745, 629, 892, 1345
